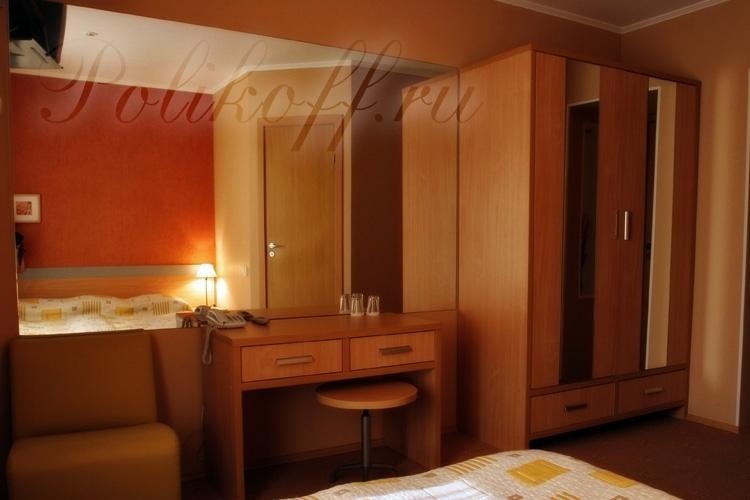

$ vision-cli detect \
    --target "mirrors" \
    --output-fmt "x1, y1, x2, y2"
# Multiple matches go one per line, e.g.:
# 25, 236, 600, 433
562, 58, 601, 383
7, 2, 458, 338
644, 69, 673, 374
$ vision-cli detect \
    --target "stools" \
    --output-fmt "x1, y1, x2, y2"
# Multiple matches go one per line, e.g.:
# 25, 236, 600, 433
314, 383, 418, 482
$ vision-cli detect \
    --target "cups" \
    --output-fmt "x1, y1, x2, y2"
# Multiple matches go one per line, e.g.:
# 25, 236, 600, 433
350, 298, 364, 316
365, 295, 380, 315
339, 292, 364, 313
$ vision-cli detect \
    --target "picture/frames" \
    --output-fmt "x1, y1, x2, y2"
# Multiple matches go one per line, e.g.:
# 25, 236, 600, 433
13, 192, 43, 224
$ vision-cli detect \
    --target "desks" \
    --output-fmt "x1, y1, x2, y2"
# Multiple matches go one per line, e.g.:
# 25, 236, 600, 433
207, 308, 444, 497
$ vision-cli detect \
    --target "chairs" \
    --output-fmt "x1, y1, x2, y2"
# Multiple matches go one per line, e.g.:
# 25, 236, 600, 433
9, 331, 182, 499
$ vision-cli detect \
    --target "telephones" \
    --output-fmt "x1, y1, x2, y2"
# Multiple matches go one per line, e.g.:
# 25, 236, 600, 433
206, 310, 246, 329
194, 305, 210, 311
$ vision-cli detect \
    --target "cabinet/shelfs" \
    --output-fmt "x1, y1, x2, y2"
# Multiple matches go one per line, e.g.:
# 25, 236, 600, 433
459, 45, 702, 452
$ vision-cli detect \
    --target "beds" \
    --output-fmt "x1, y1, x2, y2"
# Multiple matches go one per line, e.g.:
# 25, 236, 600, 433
21, 262, 216, 332
299, 447, 683, 499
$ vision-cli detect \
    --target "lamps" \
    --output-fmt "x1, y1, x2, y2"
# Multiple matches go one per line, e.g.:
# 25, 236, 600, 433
194, 263, 216, 306
